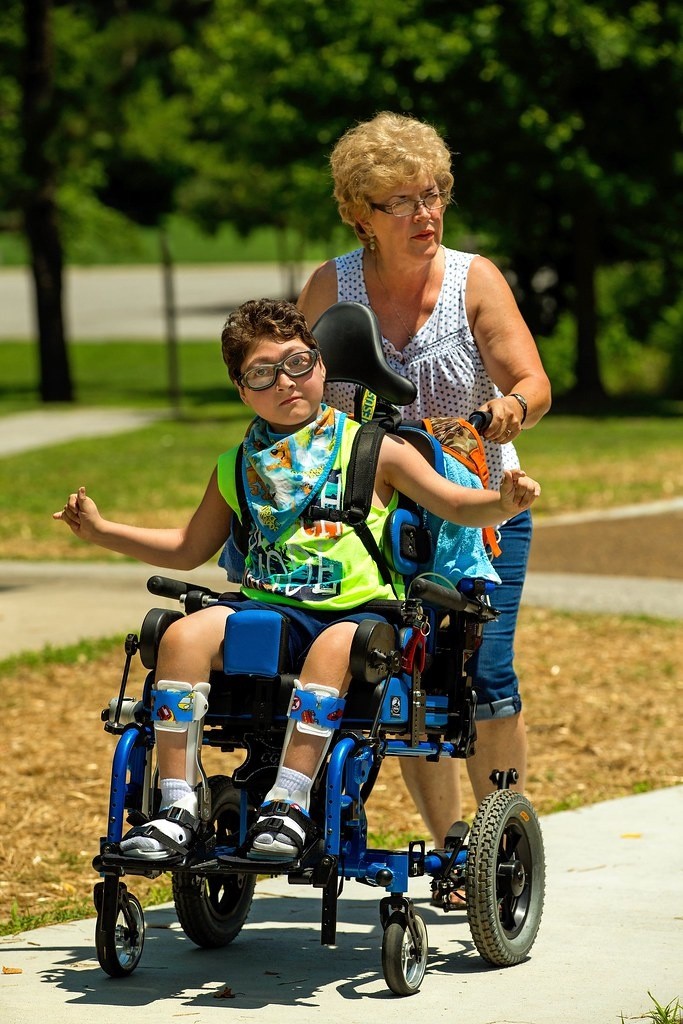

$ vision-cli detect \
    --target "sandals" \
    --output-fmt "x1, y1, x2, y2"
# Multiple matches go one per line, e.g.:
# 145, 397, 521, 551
118, 808, 203, 858
431, 885, 469, 910
245, 797, 315, 861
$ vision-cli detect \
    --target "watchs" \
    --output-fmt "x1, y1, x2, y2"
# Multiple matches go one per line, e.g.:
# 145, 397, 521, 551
509, 393, 527, 425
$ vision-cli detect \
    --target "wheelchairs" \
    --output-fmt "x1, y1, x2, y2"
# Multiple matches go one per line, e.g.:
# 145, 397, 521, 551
84, 303, 546, 993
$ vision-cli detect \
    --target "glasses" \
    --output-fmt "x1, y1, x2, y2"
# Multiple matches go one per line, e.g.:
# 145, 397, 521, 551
370, 189, 449, 218
236, 349, 320, 392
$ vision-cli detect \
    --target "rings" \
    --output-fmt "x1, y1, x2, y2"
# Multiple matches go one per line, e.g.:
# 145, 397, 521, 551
505, 430, 511, 436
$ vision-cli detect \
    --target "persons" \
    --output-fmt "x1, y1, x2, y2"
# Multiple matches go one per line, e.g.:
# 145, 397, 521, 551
52, 299, 541, 860
297, 113, 552, 907
